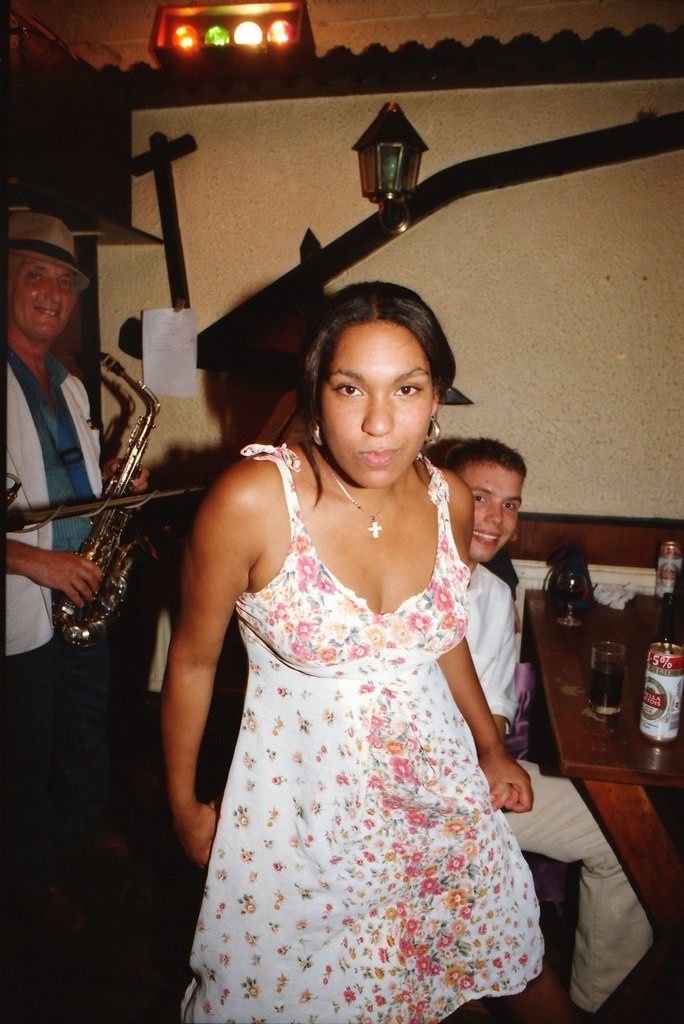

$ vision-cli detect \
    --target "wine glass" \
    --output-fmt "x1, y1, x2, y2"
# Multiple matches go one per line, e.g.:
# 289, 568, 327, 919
548, 561, 594, 626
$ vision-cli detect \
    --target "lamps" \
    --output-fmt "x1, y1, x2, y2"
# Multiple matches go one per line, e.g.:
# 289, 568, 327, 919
352, 103, 428, 234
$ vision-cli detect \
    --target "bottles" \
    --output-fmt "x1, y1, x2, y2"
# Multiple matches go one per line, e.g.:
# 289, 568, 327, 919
588, 642, 626, 715
649, 593, 680, 646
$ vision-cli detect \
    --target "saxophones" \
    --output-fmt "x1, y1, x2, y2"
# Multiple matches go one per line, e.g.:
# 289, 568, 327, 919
52, 343, 163, 653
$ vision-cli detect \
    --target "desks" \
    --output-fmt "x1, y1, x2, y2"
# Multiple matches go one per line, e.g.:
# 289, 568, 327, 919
525, 589, 684, 974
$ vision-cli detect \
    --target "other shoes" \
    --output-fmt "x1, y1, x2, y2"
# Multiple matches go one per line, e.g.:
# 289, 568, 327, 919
16, 881, 89, 941
59, 828, 133, 865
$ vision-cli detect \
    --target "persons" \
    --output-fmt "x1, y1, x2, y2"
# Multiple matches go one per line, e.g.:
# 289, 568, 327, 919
6, 210, 148, 928
162, 280, 652, 1024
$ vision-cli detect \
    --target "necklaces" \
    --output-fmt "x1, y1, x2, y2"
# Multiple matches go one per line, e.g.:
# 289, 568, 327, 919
315, 447, 393, 537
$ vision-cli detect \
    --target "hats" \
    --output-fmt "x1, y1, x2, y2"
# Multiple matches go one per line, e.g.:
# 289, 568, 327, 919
9, 211, 88, 293
